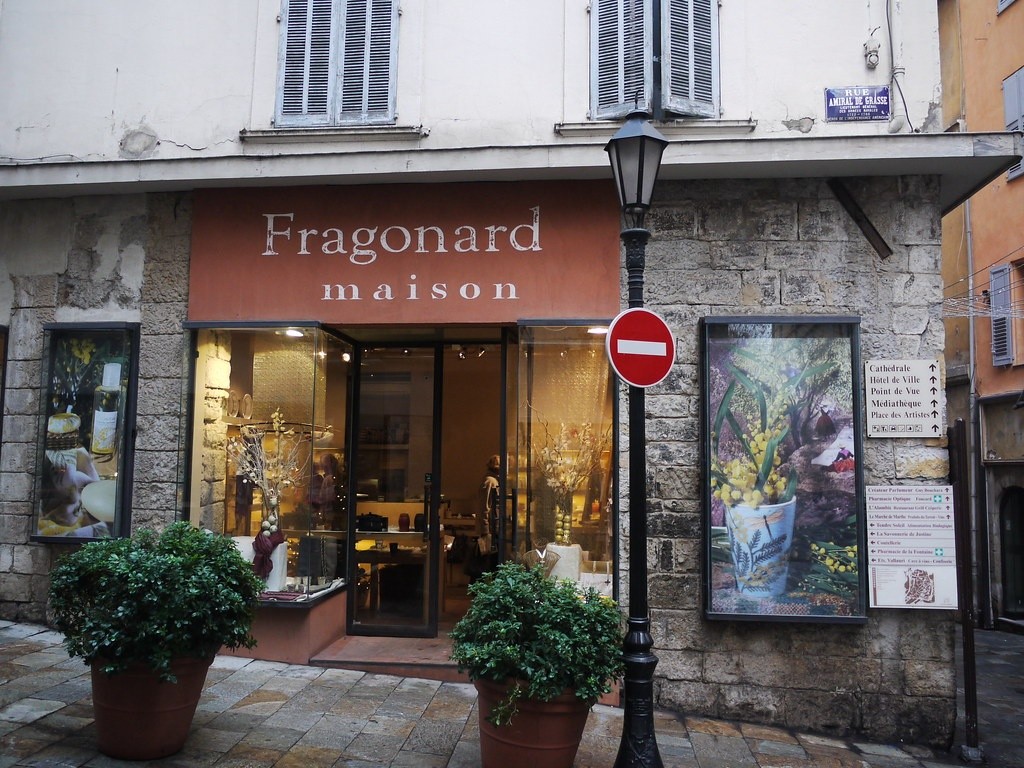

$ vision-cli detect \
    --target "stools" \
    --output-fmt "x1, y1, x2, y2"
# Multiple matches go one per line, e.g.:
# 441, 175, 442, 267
379, 564, 422, 609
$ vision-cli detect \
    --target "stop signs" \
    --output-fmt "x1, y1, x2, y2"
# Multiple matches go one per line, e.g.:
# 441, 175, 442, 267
605, 307, 677, 390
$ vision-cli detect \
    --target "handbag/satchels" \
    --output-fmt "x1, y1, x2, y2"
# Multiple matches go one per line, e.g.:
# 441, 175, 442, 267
478, 534, 498, 555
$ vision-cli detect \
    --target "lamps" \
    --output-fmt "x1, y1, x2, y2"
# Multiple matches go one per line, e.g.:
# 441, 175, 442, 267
459, 346, 467, 359
476, 346, 487, 357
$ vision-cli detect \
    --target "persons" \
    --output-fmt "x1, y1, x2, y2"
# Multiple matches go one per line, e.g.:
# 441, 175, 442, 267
319, 454, 344, 531
477, 455, 500, 573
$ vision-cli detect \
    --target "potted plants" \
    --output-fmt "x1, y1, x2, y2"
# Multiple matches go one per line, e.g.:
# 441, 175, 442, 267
447, 561, 623, 768
47, 519, 270, 762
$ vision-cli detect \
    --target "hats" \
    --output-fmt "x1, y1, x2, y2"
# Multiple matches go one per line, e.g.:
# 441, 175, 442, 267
487, 455, 501, 467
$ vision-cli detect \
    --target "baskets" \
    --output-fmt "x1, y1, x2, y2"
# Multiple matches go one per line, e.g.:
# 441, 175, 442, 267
47, 430, 79, 449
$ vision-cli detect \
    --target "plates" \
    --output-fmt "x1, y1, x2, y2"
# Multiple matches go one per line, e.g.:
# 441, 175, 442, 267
241, 393, 254, 420
227, 390, 240, 418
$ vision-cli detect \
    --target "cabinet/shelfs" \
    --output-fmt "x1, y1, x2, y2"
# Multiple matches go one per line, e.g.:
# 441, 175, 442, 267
263, 500, 480, 610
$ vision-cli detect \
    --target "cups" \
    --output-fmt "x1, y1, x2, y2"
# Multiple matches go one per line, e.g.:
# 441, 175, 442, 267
725, 496, 796, 598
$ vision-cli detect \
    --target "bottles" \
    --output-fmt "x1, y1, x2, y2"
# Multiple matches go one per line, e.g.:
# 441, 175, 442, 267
90, 363, 121, 454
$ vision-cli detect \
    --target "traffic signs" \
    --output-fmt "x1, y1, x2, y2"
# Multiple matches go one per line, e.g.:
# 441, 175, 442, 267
865, 359, 942, 439
866, 484, 958, 609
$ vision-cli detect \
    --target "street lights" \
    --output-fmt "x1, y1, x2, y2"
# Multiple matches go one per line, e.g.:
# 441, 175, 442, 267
602, 88, 668, 768
